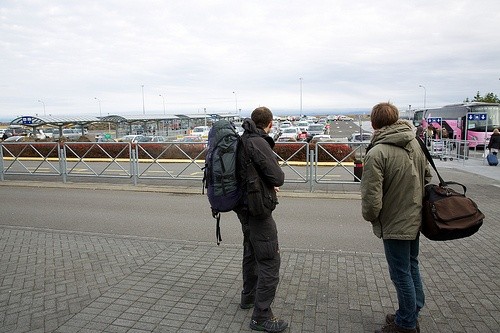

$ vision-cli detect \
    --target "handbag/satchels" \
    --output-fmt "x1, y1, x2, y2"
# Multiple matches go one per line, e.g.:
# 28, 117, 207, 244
419, 180, 486, 241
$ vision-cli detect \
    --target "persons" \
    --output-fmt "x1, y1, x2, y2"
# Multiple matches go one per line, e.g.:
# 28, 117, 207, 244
232, 106, 288, 332
488, 128, 500, 155
360, 102, 435, 333
413, 122, 448, 146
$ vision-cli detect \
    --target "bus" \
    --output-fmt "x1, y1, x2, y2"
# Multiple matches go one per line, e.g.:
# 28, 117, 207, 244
52, 129, 88, 142
413, 102, 499, 149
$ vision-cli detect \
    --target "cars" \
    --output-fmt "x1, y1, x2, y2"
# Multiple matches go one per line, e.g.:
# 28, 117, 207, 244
0, 127, 53, 155
233, 116, 332, 147
328, 115, 353, 121
89, 125, 208, 153
348, 133, 372, 154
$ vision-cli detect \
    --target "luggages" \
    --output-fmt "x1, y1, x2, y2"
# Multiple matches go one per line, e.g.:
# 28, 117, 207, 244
487, 147, 498, 166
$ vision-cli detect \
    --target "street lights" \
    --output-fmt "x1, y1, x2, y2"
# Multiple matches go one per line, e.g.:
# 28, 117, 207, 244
94, 97, 102, 122
38, 100, 46, 121
140, 84, 145, 116
419, 85, 426, 108
203, 107, 207, 131
298, 77, 302, 114
159, 94, 165, 115
232, 92, 238, 113
238, 109, 241, 127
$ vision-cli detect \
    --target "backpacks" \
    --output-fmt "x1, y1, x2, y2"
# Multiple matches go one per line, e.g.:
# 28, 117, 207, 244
202, 119, 263, 212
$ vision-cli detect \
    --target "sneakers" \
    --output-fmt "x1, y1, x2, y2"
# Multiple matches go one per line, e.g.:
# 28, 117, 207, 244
240, 301, 255, 309
250, 315, 288, 333
374, 323, 418, 333
387, 312, 420, 333
